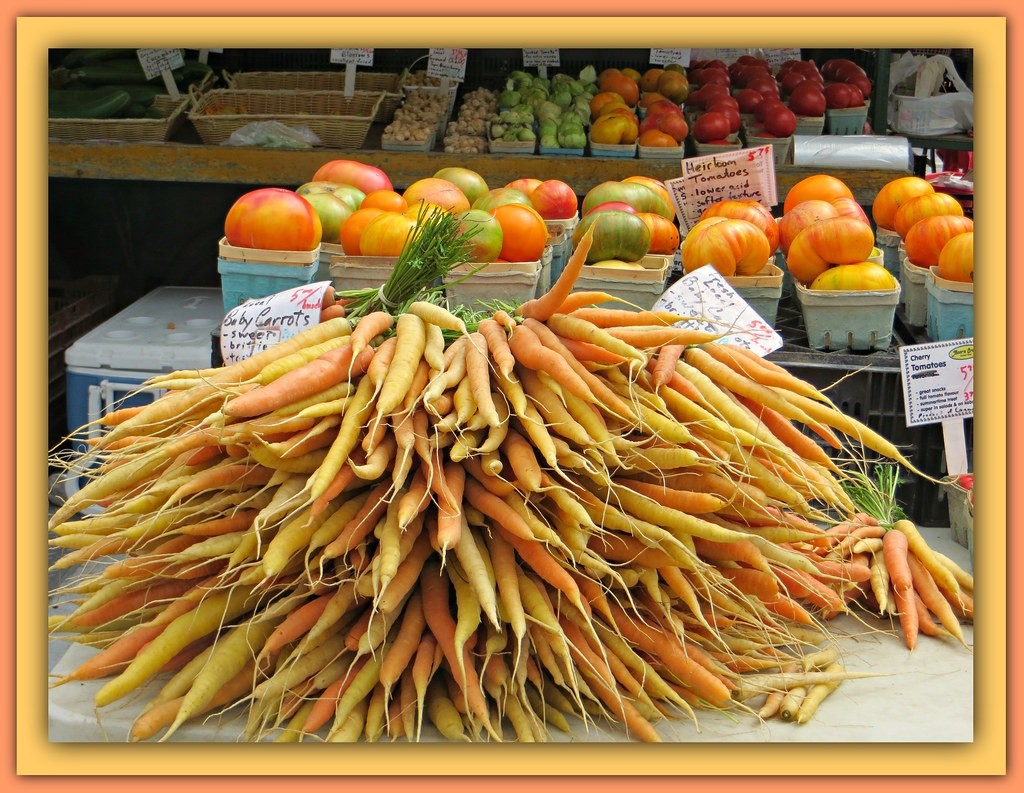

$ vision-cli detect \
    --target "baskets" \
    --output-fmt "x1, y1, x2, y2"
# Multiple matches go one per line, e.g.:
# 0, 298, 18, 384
48, 67, 409, 154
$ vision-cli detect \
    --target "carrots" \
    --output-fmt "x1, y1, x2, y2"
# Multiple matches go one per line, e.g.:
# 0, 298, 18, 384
48, 213, 974, 743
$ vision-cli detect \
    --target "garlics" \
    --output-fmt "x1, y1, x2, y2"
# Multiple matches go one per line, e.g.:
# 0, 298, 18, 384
381, 69, 498, 154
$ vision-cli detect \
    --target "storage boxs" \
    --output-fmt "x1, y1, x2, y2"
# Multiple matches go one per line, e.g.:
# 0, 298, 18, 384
216, 236, 322, 314
867, 247, 885, 267
926, 267, 974, 341
897, 239, 907, 303
568, 216, 795, 329
875, 226, 900, 275
903, 257, 928, 327
943, 473, 974, 550
380, 73, 792, 163
329, 253, 398, 292
64, 286, 223, 515
962, 488, 974, 573
535, 243, 554, 298
547, 225, 568, 286
795, 275, 902, 351
441, 258, 543, 310
826, 104, 868, 135
795, 113, 826, 136
318, 243, 343, 279
543, 210, 578, 257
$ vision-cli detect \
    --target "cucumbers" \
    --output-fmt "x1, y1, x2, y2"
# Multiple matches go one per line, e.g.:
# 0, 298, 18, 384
48, 48, 212, 120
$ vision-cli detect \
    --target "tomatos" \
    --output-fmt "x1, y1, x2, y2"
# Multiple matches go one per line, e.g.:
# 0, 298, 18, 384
224, 161, 975, 291
591, 63, 690, 148
685, 55, 870, 145
